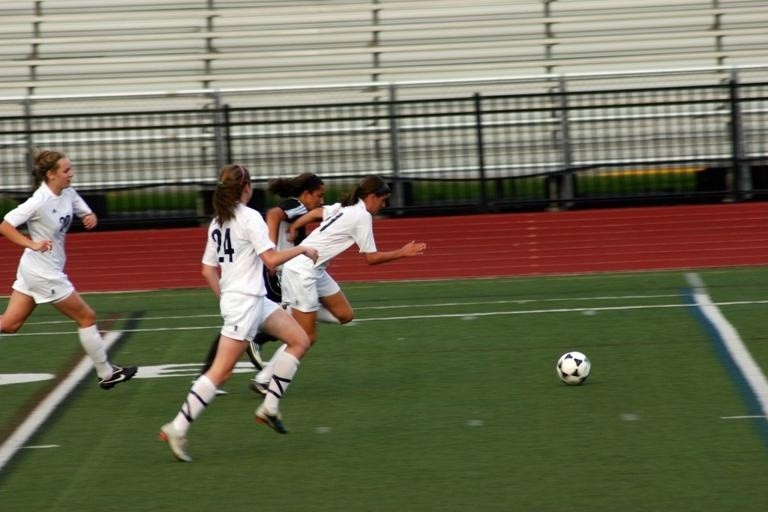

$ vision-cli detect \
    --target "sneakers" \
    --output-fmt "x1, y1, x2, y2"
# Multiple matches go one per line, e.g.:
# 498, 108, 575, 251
97, 363, 137, 390
189, 374, 230, 397
247, 376, 270, 397
158, 422, 193, 462
254, 404, 289, 434
246, 340, 265, 372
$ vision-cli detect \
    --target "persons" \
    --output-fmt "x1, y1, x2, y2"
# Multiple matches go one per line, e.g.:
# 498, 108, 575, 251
1, 145, 139, 391
157, 163, 321, 463
187, 172, 328, 398
247, 174, 428, 398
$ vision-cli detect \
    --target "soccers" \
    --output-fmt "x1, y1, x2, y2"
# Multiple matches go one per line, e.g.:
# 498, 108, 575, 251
557, 351, 592, 385
